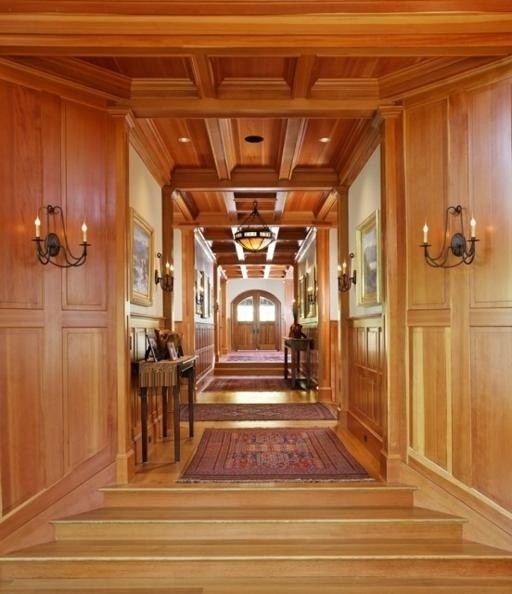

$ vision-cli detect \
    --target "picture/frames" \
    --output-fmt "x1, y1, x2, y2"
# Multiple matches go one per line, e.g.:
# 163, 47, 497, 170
126, 205, 155, 306
296, 274, 306, 318
144, 327, 163, 362
355, 206, 384, 307
167, 340, 178, 360
193, 269, 214, 319
306, 266, 317, 318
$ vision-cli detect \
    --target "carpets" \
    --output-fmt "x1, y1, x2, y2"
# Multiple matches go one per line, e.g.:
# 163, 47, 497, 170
175, 427, 376, 484
202, 378, 318, 392
219, 350, 292, 364
178, 402, 337, 421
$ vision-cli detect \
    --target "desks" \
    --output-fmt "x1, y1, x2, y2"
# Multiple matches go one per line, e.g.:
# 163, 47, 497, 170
137, 355, 197, 462
282, 336, 313, 389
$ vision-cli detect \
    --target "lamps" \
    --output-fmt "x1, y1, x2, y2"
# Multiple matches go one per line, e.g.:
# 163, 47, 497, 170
233, 201, 276, 252
153, 257, 175, 293
419, 204, 480, 268
31, 202, 88, 267
336, 252, 357, 292
